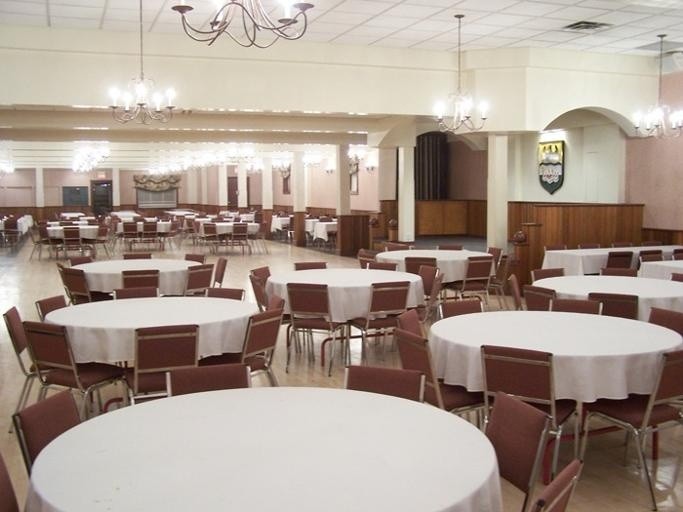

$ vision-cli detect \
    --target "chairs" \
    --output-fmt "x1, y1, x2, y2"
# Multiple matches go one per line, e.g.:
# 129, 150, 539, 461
4, 243, 683, 512
4, 204, 269, 265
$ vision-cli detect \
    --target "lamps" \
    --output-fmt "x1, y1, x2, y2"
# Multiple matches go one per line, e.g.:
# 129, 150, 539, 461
106, 1, 177, 128
432, 13, 490, 132
168, 0, 314, 46
0, 140, 377, 184
628, 33, 682, 138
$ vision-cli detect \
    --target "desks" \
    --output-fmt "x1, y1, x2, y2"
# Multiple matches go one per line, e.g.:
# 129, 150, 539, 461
272, 215, 338, 251
